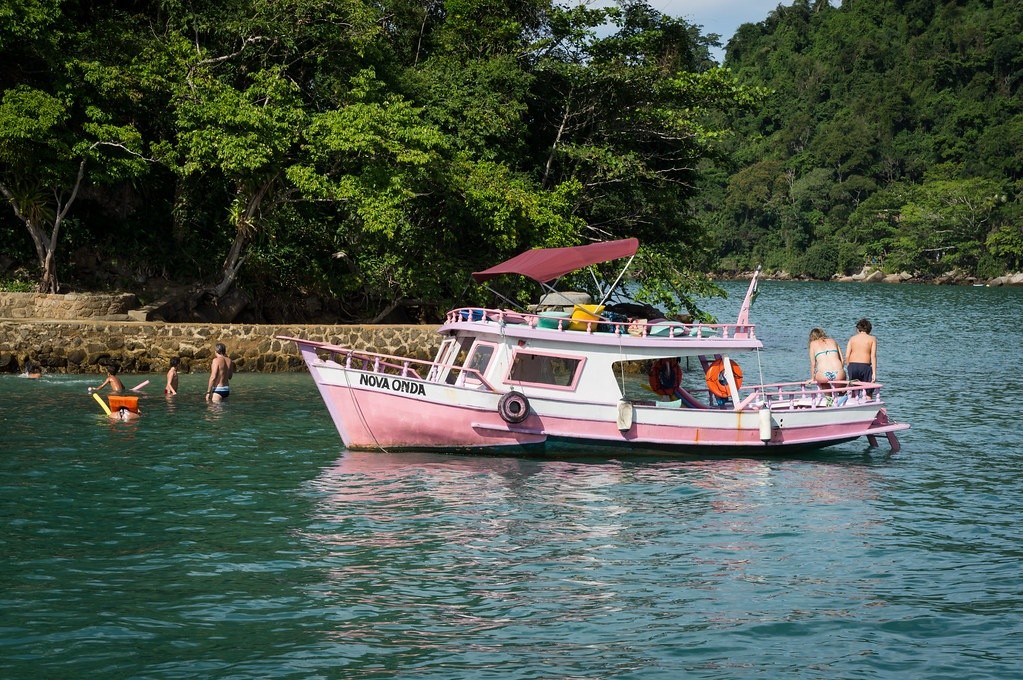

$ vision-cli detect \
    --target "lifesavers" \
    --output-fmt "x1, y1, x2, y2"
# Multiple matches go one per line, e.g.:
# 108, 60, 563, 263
92, 392, 111, 415
706, 359, 743, 398
649, 359, 682, 395
497, 391, 530, 424
87, 379, 150, 391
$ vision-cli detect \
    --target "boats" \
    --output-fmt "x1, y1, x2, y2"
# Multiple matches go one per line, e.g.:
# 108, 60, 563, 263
275, 262, 913, 462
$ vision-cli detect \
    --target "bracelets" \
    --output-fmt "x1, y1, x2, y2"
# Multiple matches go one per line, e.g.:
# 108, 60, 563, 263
206, 392, 210, 394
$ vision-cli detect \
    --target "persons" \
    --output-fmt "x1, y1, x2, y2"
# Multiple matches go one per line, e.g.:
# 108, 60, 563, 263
164, 358, 181, 396
805, 328, 846, 397
26, 365, 42, 379
206, 343, 233, 404
90, 366, 125, 391
844, 319, 877, 401
